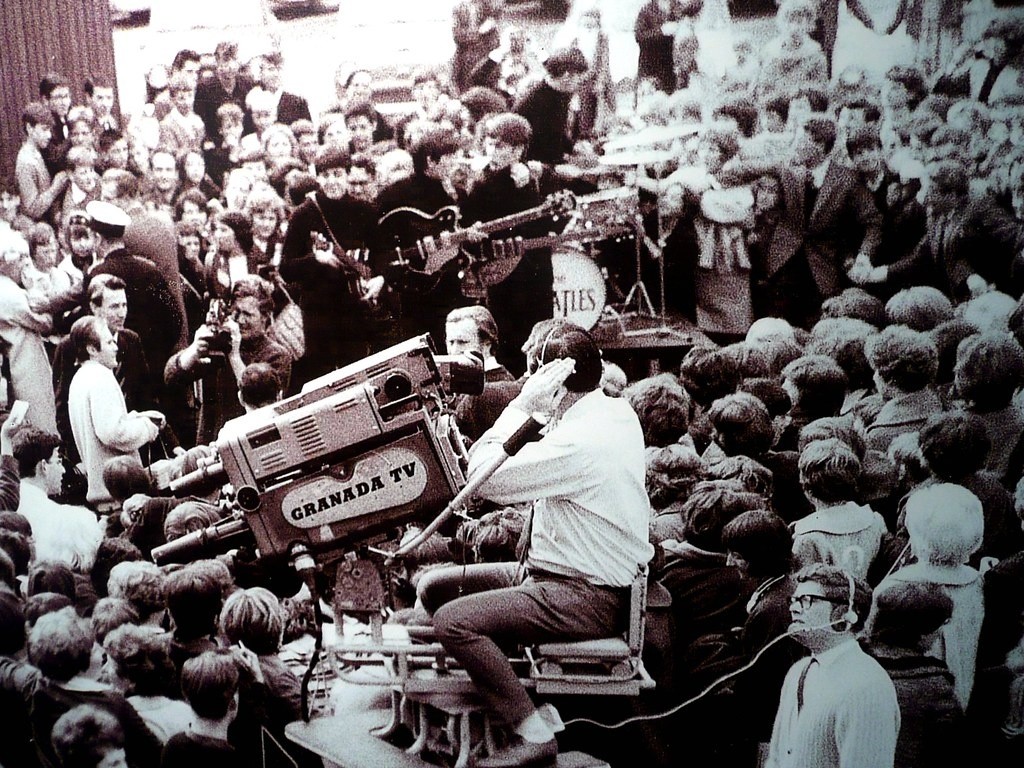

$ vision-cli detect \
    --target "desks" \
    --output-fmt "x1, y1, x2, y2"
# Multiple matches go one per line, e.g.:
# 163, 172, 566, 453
592, 311, 722, 377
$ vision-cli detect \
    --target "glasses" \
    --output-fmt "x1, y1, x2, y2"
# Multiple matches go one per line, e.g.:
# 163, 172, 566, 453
786, 594, 829, 611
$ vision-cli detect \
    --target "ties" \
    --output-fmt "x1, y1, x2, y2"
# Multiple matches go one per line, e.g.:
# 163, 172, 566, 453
797, 657, 818, 712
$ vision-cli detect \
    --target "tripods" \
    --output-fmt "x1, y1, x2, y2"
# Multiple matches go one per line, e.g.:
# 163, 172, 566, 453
616, 164, 693, 343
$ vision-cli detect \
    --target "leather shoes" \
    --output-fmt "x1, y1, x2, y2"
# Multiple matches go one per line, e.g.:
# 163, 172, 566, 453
474, 732, 558, 768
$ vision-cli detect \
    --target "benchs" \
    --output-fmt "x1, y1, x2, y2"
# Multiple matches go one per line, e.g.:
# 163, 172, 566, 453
533, 554, 648, 698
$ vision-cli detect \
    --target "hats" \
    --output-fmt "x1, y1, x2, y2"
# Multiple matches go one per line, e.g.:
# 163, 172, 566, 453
86, 201, 127, 240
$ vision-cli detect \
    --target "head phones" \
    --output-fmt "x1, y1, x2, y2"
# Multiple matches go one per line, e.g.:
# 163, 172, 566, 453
829, 565, 858, 631
537, 326, 563, 373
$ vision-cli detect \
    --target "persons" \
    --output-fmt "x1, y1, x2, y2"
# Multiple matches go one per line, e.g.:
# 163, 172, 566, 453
418, 320, 648, 768
764, 564, 901, 768
0, 0, 1024, 768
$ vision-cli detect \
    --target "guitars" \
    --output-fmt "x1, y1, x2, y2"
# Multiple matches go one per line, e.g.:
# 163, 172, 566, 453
458, 211, 638, 288
375, 187, 578, 296
